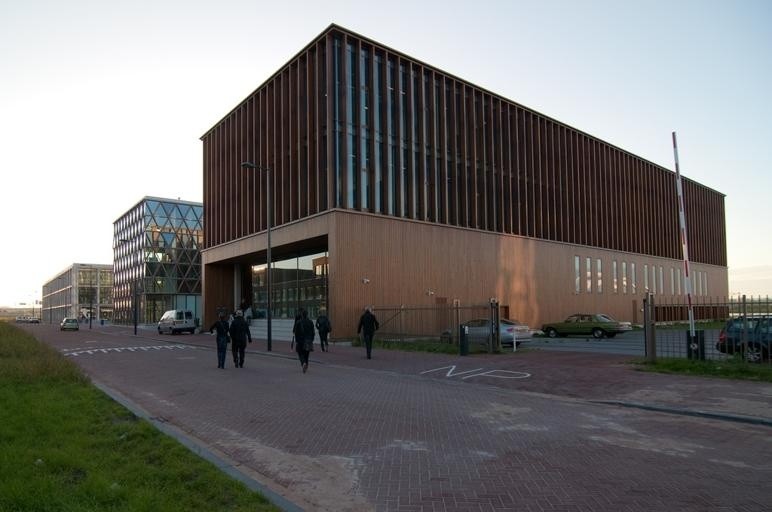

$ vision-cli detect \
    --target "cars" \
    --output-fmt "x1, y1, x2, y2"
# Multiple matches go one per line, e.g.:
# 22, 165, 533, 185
15, 316, 38, 323
440, 318, 533, 347
542, 313, 633, 339
60, 318, 78, 331
717, 316, 772, 363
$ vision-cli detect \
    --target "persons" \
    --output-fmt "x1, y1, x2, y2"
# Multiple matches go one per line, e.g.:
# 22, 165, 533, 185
293, 309, 310, 332
316, 311, 332, 352
229, 310, 251, 368
357, 307, 379, 359
210, 312, 229, 369
295, 311, 315, 374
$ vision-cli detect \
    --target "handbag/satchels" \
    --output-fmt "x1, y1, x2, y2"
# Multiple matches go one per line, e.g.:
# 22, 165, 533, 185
324, 320, 332, 332
351, 335, 361, 346
303, 340, 315, 352
221, 334, 230, 343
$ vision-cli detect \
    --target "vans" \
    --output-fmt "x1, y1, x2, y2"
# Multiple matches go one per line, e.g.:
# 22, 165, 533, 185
158, 309, 196, 335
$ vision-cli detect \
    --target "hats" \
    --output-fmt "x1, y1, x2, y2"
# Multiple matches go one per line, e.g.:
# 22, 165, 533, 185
235, 310, 243, 316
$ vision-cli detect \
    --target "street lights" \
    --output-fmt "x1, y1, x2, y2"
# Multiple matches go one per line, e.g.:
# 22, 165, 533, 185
119, 239, 138, 335
59, 277, 67, 317
44, 286, 52, 324
80, 263, 92, 329
242, 160, 272, 353
